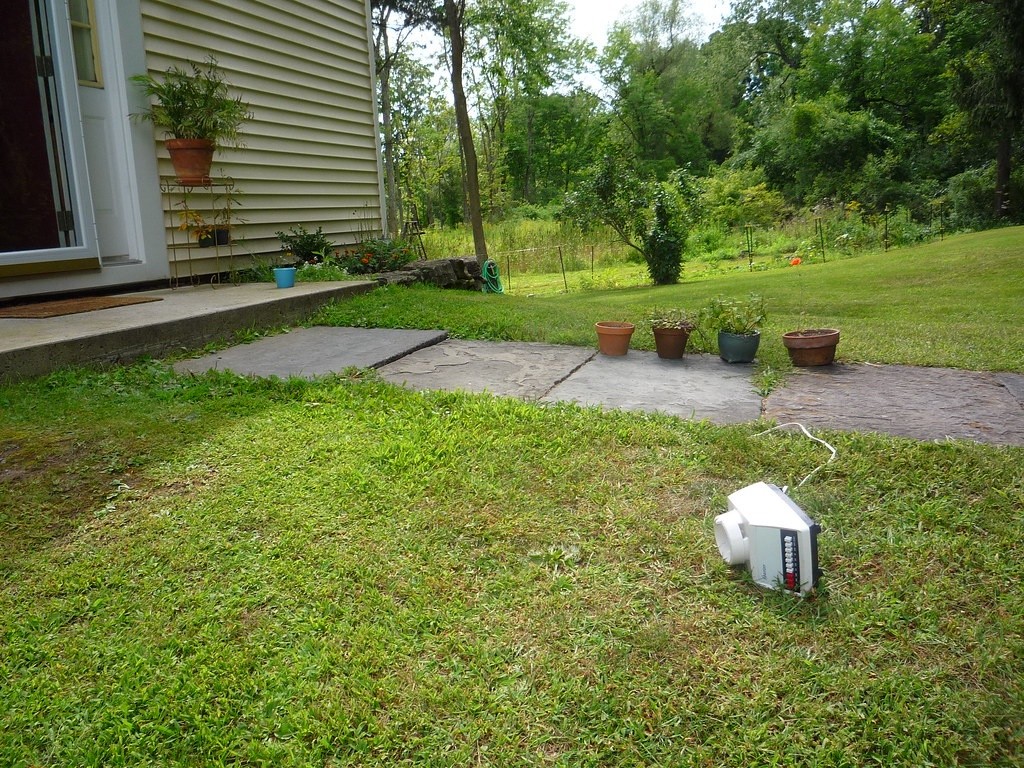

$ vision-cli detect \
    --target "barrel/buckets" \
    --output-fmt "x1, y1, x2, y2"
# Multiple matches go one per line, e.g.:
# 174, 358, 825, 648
273, 268, 297, 288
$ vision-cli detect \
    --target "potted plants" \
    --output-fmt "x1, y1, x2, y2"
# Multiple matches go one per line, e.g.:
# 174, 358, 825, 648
704, 292, 767, 362
125, 48, 255, 184
173, 193, 246, 248
651, 303, 693, 359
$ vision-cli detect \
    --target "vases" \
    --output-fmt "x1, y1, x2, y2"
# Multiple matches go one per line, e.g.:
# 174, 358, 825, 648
595, 322, 635, 356
273, 268, 296, 289
782, 326, 841, 367
